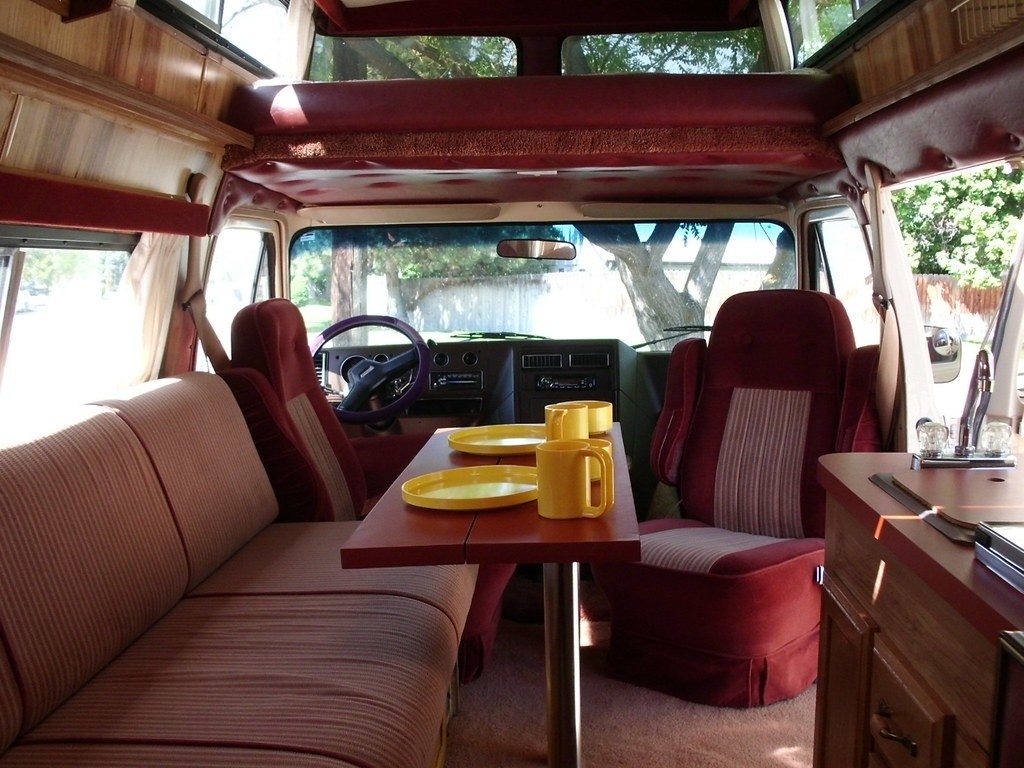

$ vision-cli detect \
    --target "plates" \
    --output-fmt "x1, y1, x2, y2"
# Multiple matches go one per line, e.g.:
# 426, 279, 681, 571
400, 464, 538, 510
447, 424, 547, 455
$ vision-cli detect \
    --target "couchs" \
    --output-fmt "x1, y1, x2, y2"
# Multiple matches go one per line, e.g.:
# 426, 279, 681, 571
590, 289, 878, 709
217, 298, 517, 684
0, 372, 479, 768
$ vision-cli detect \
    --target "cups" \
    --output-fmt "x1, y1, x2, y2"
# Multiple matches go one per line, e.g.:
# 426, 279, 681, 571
535, 441, 614, 519
917, 421, 949, 457
981, 421, 1014, 458
545, 403, 589, 442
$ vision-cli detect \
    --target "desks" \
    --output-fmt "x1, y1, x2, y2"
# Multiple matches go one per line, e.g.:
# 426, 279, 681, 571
341, 422, 640, 768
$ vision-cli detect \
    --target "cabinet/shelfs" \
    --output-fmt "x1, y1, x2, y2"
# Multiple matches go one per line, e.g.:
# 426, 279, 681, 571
813, 453, 1024, 768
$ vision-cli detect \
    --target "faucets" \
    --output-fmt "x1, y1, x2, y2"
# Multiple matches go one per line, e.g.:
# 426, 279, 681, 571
955, 349, 994, 455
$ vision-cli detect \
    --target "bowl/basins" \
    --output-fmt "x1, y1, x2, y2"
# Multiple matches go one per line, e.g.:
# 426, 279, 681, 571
577, 438, 614, 481
577, 400, 614, 436
